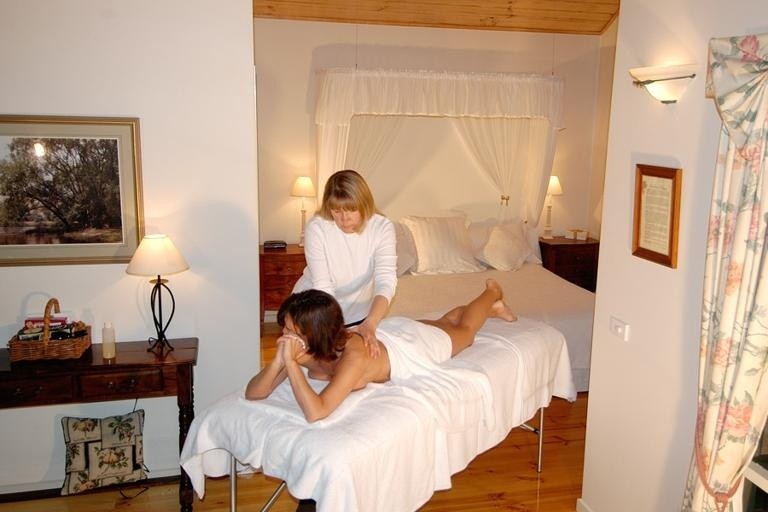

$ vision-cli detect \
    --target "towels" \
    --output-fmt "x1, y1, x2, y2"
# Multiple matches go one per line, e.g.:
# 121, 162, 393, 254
239, 367, 384, 429
347, 317, 496, 437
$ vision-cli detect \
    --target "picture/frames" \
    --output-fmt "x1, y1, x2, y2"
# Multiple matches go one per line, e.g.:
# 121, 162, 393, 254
631, 164, 682, 268
0, 115, 144, 266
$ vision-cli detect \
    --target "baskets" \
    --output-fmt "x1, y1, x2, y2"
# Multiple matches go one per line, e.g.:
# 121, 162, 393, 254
9, 298, 92, 363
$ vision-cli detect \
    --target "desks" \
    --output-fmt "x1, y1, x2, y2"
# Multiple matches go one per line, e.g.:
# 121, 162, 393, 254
1, 339, 198, 512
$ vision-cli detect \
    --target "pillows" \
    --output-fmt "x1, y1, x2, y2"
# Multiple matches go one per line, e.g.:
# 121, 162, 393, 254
392, 216, 543, 278
61, 409, 147, 495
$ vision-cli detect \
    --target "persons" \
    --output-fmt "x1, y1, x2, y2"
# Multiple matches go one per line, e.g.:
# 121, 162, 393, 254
246, 277, 518, 424
292, 169, 398, 358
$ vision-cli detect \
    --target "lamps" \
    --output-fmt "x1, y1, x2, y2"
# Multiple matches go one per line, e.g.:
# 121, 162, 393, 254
543, 176, 563, 239
290, 177, 317, 247
126, 234, 190, 350
629, 63, 697, 103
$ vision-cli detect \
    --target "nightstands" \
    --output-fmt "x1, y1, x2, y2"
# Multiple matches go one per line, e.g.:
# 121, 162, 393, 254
539, 236, 600, 293
260, 244, 307, 327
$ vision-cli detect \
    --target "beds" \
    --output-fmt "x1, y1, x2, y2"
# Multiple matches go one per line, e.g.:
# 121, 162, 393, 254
178, 307, 578, 512
385, 263, 595, 392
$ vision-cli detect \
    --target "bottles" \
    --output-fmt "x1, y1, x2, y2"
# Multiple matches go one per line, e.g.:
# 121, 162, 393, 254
101, 321, 115, 360
